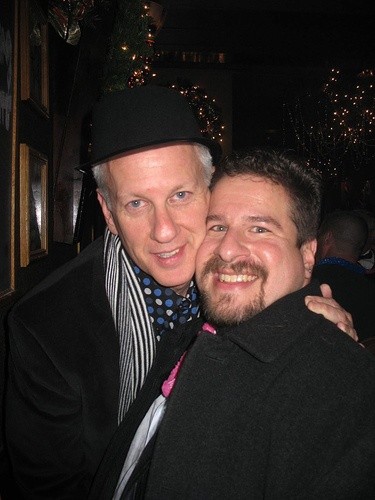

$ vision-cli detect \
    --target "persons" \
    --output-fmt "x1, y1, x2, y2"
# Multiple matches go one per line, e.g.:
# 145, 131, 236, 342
311, 119, 374, 356
98, 149, 375, 500
0, 82, 367, 500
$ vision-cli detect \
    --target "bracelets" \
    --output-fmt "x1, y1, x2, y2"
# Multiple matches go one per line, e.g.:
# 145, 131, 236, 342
354, 337, 364, 345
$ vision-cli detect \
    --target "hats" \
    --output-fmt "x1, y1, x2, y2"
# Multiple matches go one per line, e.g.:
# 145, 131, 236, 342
75, 83, 222, 181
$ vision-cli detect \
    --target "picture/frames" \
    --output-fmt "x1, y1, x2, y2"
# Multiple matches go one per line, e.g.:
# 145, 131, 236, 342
19, 144, 52, 268
0, 0, 19, 299
19, 0, 50, 120
